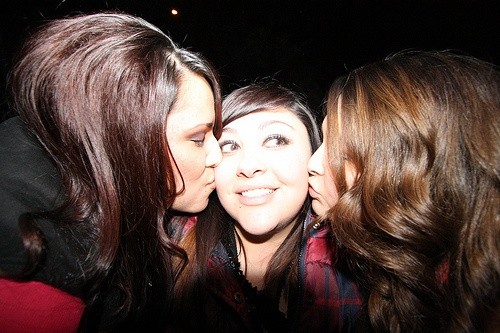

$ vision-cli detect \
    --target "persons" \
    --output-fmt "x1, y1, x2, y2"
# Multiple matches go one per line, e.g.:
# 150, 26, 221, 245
0, 11, 223, 333
306, 49, 500, 333
164, 79, 363, 333
